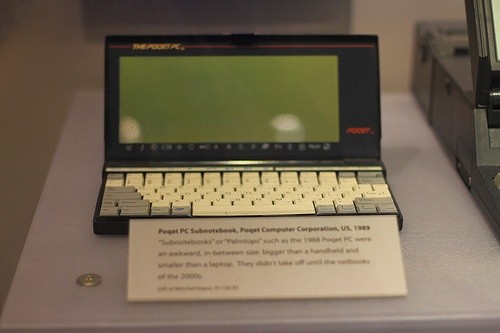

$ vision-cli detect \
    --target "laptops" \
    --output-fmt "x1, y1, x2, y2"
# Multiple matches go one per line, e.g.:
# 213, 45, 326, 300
91, 32, 405, 235
